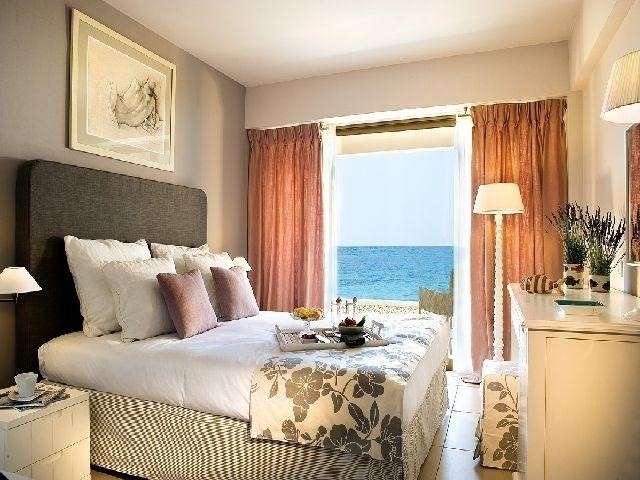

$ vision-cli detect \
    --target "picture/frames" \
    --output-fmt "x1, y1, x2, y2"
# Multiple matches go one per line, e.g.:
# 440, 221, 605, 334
68, 9, 176, 171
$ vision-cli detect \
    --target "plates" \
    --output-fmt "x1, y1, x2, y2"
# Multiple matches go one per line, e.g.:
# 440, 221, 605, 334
552, 299, 607, 316
8, 391, 38, 402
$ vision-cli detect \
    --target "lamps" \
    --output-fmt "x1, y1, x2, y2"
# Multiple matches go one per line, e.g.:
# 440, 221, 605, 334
0, 265, 42, 302
231, 256, 253, 272
473, 183, 525, 361
601, 50, 640, 126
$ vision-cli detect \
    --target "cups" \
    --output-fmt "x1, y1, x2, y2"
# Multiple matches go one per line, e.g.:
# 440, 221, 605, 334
14, 371, 38, 398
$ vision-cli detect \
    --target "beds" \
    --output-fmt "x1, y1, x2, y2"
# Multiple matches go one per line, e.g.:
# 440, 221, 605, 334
15, 159, 450, 479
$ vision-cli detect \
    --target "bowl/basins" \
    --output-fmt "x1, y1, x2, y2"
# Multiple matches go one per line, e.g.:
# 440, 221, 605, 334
339, 326, 364, 336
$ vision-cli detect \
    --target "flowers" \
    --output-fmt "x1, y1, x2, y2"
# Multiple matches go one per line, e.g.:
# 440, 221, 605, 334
546, 203, 587, 262
579, 206, 626, 273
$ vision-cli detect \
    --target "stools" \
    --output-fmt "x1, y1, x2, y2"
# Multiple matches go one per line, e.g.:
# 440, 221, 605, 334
480, 378, 519, 470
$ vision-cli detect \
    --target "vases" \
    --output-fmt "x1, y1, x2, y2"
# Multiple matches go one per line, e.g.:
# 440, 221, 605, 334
561, 262, 581, 288
589, 273, 610, 292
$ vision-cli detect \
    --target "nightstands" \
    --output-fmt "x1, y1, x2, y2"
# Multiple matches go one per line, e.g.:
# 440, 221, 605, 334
0, 381, 91, 480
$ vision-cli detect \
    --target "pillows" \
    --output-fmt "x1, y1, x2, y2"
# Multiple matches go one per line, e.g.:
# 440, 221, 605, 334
182, 249, 232, 311
64, 234, 151, 335
208, 265, 259, 320
157, 268, 218, 337
151, 242, 209, 265
106, 253, 177, 343
